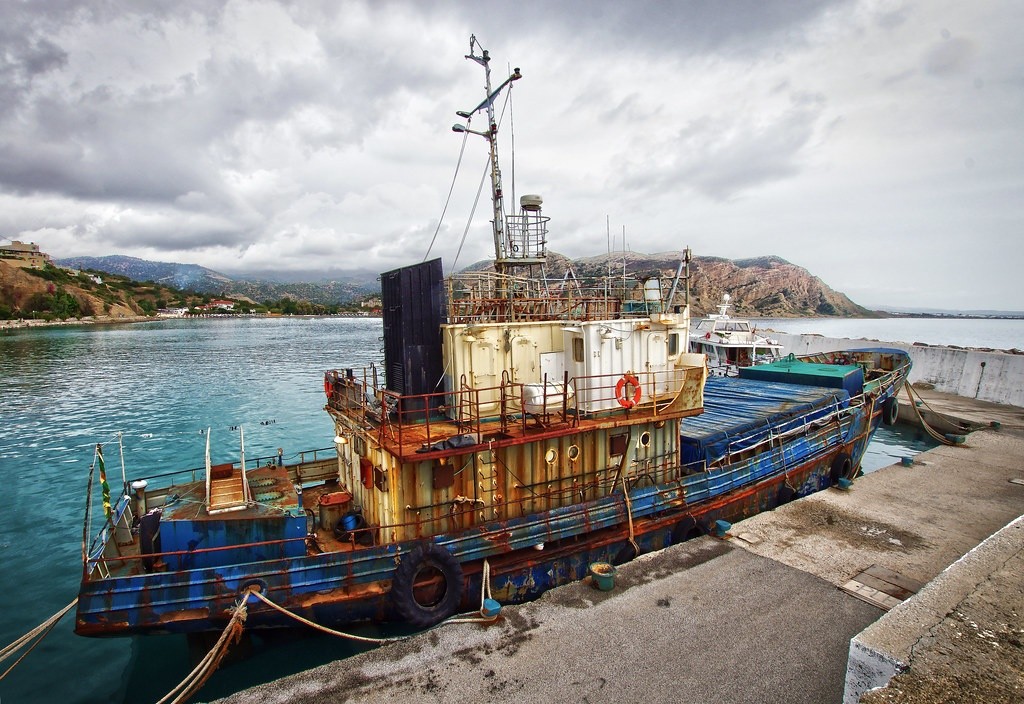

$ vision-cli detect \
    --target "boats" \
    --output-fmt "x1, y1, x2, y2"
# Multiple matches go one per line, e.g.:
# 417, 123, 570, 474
71, 32, 914, 660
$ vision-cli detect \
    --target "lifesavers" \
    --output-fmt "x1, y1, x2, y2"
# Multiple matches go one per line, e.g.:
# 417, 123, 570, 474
672, 518, 708, 544
706, 333, 710, 340
395, 542, 465, 630
615, 375, 642, 409
324, 374, 334, 399
831, 453, 853, 483
882, 396, 899, 426
776, 486, 799, 506
614, 544, 651, 566
